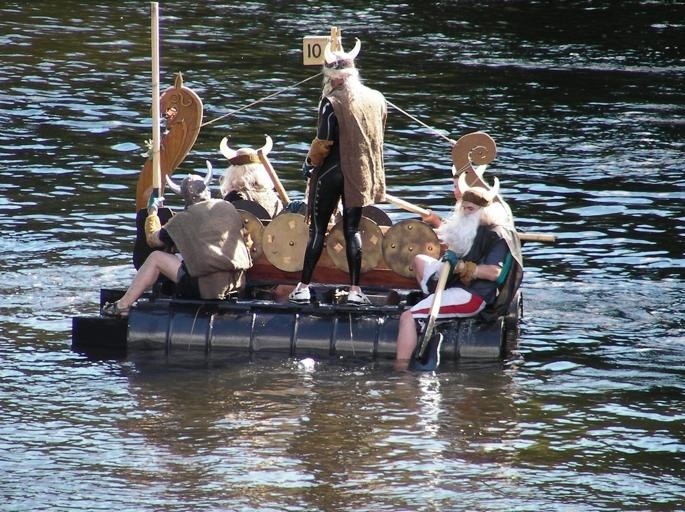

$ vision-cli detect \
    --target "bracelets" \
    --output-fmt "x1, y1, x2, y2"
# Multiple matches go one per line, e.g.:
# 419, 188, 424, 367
459, 260, 478, 286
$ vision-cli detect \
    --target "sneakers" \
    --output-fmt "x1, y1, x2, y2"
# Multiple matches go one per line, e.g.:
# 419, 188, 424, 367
346, 290, 373, 305
287, 280, 311, 305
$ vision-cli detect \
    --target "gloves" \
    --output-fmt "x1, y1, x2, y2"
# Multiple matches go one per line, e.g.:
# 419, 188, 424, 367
301, 155, 315, 178
144, 190, 165, 216
440, 249, 461, 271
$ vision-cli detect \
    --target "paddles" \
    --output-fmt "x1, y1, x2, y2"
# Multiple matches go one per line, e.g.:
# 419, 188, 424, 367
517, 233, 584, 245
133, 1, 175, 271
407, 260, 451, 373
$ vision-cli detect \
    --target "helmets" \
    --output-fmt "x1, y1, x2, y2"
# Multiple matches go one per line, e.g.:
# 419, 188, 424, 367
229, 147, 264, 166
321, 50, 359, 78
460, 186, 491, 208
178, 173, 207, 199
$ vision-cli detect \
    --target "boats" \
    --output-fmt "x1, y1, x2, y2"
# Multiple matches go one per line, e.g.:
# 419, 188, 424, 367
73, 0, 523, 358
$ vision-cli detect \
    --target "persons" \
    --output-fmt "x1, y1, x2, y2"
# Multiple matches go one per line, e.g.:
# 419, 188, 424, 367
288, 38, 388, 304
395, 170, 524, 372
98, 157, 254, 317
215, 135, 289, 302
421, 162, 488, 260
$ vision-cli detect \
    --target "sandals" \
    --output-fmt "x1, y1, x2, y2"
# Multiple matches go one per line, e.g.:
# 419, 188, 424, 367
98, 301, 130, 320
